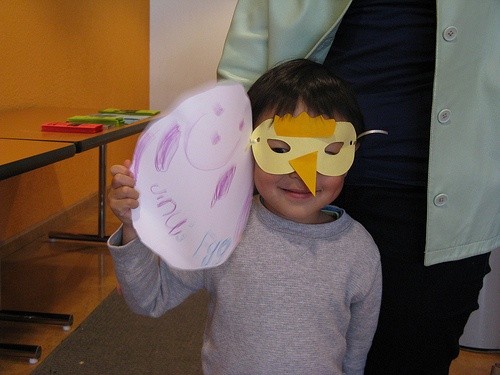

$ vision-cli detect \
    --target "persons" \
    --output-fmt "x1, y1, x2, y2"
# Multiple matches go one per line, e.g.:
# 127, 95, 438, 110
216, 1, 500, 375
108, 59, 382, 375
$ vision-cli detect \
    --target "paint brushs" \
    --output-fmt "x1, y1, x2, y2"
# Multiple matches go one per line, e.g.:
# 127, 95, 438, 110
39, 107, 161, 136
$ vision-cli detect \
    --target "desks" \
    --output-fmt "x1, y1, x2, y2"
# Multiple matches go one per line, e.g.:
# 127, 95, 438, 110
0, 115, 159, 359
